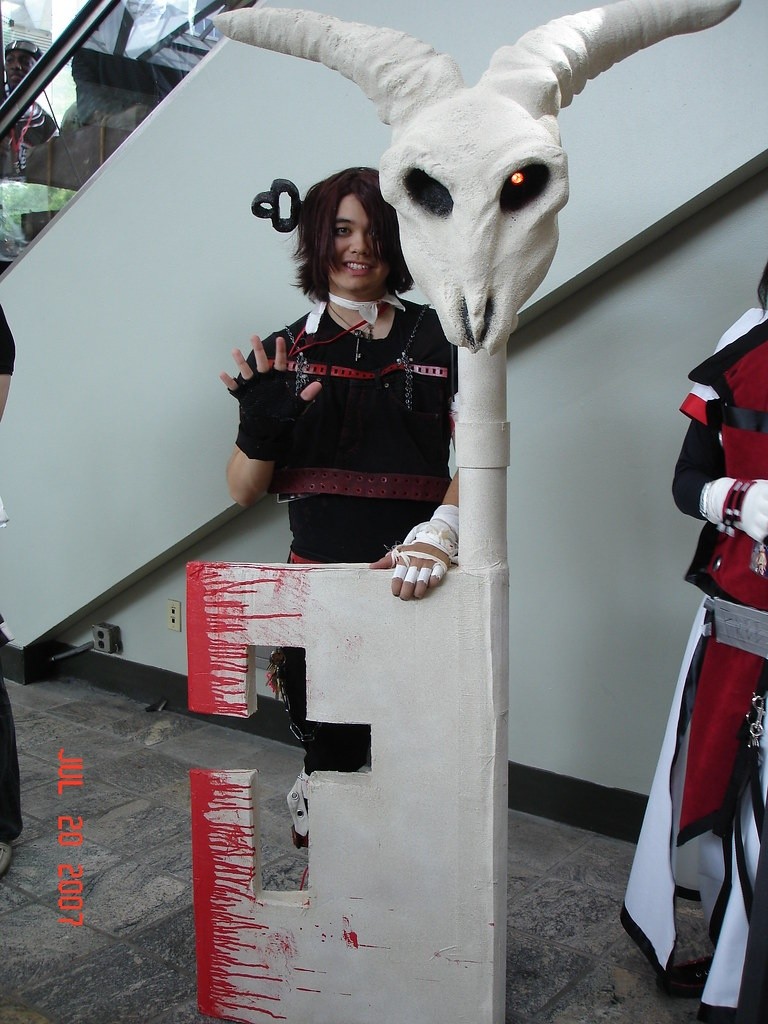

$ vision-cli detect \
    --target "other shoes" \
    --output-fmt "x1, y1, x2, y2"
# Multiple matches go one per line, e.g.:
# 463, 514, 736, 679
656, 955, 717, 998
0, 839, 14, 875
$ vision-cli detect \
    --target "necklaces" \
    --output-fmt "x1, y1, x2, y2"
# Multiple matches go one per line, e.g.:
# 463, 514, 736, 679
326, 304, 389, 360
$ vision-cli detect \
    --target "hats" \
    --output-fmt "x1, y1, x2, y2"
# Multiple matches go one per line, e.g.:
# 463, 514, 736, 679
5, 40, 43, 62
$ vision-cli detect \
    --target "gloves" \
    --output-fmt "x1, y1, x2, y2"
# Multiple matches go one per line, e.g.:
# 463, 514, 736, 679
700, 477, 768, 546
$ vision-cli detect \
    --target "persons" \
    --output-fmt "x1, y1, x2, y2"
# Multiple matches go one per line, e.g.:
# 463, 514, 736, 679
221, 166, 459, 850
619, 259, 768, 1024
0, 305, 24, 875
0, 39, 59, 177
